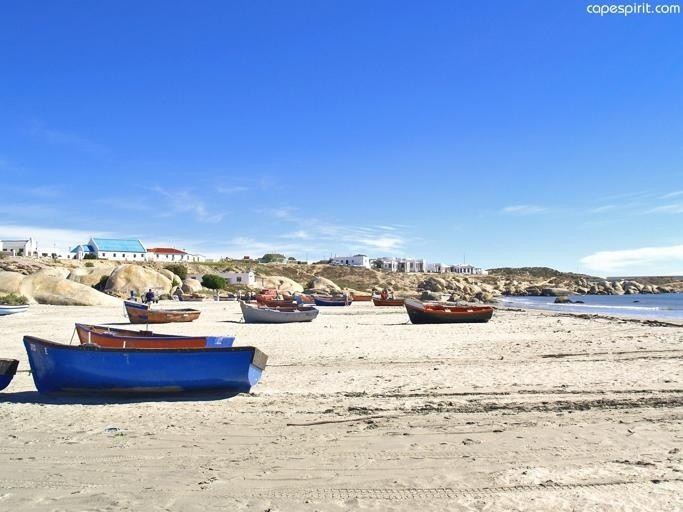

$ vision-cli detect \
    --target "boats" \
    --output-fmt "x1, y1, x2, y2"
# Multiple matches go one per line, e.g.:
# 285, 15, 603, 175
124, 301, 201, 323
240, 300, 319, 323
213, 287, 372, 306
75, 323, 235, 349
373, 298, 405, 306
405, 301, 493, 324
24, 335, 268, 392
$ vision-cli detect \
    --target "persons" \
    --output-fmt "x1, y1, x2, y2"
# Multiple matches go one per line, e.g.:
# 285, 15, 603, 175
236, 289, 241, 302
245, 290, 255, 300
380, 289, 396, 299
146, 289, 154, 310
